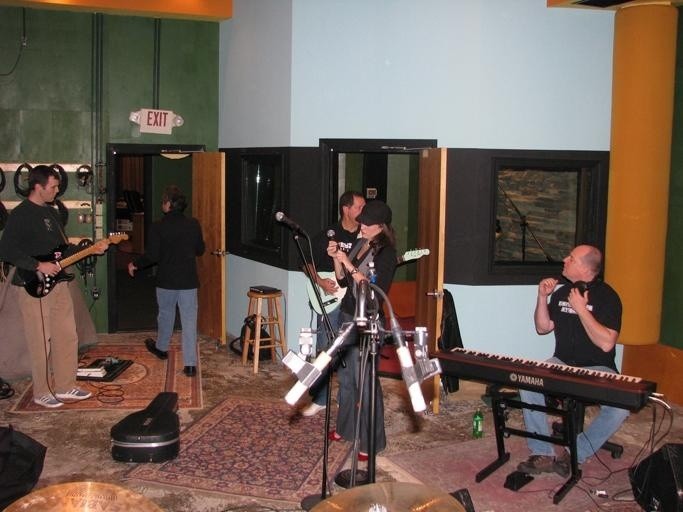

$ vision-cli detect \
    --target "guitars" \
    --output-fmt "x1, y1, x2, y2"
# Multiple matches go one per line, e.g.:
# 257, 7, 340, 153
22, 231, 129, 297
306, 247, 430, 315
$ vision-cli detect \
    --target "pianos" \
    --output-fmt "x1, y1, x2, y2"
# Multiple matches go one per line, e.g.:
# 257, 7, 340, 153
428, 346, 656, 414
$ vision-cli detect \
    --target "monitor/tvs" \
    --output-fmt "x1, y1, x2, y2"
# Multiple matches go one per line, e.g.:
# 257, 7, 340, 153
124, 191, 143, 212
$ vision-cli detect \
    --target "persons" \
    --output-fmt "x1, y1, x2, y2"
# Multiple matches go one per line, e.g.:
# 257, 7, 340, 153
294, 191, 369, 416
0, 165, 111, 410
126, 186, 206, 376
517, 243, 630, 477
326, 201, 397, 462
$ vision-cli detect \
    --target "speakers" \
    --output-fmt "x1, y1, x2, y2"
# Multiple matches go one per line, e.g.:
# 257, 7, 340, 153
628, 442, 683, 512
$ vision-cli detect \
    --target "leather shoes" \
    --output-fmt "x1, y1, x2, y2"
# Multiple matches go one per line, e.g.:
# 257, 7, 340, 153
328, 430, 342, 441
358, 452, 368, 461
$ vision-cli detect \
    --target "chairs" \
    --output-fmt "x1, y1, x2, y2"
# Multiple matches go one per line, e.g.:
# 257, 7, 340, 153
544, 343, 627, 471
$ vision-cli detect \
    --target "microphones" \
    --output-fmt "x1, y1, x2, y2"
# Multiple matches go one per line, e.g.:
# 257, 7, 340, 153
388, 317, 429, 413
282, 320, 355, 408
276, 211, 305, 236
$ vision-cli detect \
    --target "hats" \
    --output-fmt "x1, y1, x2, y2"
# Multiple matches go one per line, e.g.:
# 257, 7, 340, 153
355, 200, 392, 226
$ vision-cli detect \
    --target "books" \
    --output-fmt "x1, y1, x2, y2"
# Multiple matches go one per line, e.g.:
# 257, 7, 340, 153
76, 363, 108, 379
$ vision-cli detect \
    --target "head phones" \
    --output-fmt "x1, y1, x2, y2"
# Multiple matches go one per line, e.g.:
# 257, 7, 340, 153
77, 164, 93, 186
573, 274, 605, 293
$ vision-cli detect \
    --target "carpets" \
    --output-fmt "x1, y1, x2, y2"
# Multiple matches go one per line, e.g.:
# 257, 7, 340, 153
383, 422, 659, 512
124, 392, 355, 504
9, 342, 204, 409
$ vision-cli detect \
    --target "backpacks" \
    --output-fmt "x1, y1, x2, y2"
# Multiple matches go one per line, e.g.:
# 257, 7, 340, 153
230, 323, 272, 360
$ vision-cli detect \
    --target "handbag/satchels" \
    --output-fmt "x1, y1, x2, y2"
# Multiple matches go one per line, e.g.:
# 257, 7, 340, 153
0, 424, 47, 511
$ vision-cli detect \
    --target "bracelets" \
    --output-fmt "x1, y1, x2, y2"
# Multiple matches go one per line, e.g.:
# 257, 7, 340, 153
349, 267, 359, 275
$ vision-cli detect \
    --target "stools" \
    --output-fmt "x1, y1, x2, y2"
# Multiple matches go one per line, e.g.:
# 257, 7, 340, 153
242, 290, 290, 372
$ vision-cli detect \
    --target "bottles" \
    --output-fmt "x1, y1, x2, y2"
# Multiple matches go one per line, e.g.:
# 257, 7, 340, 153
472, 406, 483, 438
366, 261, 378, 285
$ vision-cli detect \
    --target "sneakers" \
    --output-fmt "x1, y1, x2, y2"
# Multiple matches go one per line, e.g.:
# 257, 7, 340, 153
34, 394, 64, 408
184, 366, 196, 376
555, 452, 571, 477
299, 401, 326, 416
146, 338, 168, 359
518, 455, 554, 474
56, 389, 92, 400
